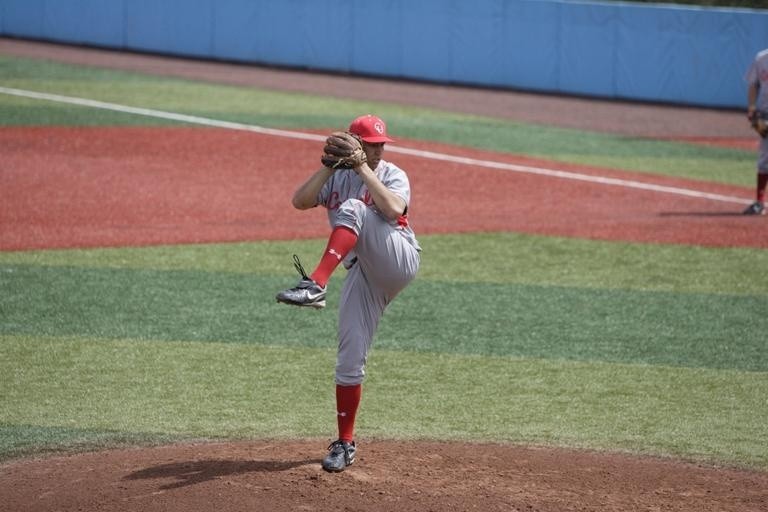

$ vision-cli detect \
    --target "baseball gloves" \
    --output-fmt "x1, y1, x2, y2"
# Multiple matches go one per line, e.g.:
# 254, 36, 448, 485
748, 110, 767, 139
321, 131, 367, 169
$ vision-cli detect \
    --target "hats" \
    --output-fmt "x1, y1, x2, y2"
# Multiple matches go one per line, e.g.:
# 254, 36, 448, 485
350, 115, 395, 143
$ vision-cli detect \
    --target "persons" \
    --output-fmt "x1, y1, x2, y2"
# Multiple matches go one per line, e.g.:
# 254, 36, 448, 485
276, 116, 423, 473
745, 48, 768, 217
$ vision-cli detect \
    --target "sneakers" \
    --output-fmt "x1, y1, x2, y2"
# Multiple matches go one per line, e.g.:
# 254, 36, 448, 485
322, 440, 356, 471
744, 203, 766, 216
276, 255, 327, 308
